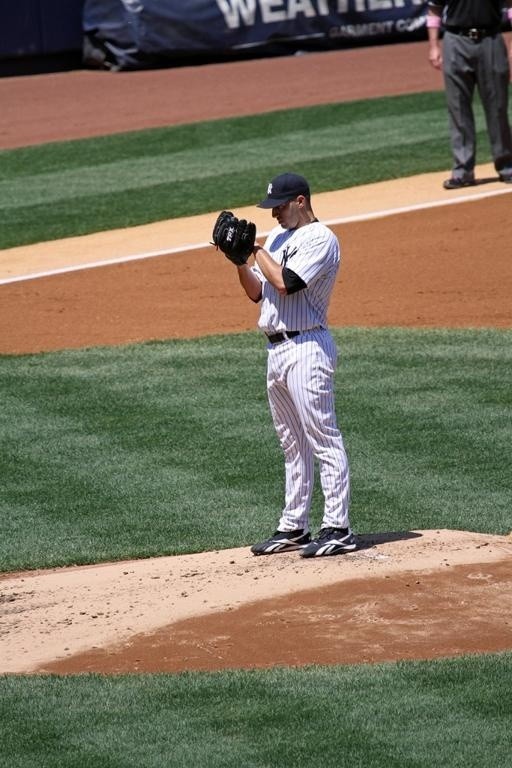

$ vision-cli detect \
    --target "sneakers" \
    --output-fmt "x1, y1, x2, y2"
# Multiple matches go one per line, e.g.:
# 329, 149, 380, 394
248, 528, 312, 555
442, 172, 511, 190
298, 524, 358, 558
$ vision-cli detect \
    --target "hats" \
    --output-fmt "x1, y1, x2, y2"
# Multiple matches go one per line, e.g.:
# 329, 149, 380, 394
256, 173, 311, 209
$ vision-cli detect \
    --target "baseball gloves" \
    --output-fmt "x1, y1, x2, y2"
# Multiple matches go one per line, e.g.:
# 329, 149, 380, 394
212, 211, 255, 266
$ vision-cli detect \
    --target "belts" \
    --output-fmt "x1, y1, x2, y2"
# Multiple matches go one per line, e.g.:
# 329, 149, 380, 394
443, 21, 499, 41
264, 331, 300, 344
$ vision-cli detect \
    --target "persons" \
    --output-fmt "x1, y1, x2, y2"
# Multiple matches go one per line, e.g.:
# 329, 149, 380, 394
207, 172, 357, 558
425, 1, 512, 189
